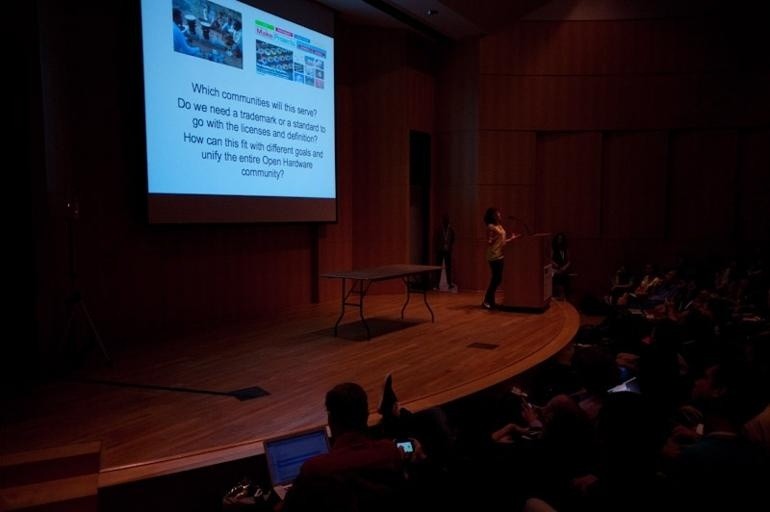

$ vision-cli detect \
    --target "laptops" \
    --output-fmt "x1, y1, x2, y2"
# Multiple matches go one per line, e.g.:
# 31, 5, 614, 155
263, 423, 330, 501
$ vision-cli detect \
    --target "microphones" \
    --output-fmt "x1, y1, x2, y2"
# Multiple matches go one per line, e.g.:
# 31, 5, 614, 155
508, 214, 534, 236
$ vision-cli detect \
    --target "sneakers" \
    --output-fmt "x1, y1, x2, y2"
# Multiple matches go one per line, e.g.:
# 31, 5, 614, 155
376, 373, 397, 414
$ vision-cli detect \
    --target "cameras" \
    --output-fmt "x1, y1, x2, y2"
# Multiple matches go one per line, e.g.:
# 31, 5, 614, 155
396, 440, 415, 454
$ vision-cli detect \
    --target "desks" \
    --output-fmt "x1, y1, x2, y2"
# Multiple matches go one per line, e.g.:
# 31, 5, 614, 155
319, 262, 442, 337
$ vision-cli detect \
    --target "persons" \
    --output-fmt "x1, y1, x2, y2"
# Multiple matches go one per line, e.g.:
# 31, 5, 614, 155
591, 262, 770, 511
479, 206, 522, 309
432, 213, 457, 291
551, 231, 574, 299
278, 374, 590, 511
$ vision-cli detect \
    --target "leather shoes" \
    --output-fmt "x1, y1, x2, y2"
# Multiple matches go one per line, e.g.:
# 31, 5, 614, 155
482, 301, 491, 309
